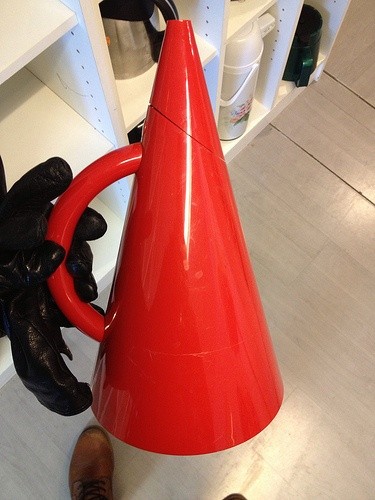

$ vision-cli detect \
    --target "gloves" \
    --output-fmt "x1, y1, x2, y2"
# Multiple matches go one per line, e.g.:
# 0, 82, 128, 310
2, 159, 107, 304
9, 270, 107, 415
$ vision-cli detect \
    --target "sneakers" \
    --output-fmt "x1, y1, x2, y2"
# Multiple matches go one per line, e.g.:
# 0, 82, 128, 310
67, 426, 115, 498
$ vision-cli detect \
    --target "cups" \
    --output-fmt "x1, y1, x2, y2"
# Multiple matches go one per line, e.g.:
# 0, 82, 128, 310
282, 3, 323, 88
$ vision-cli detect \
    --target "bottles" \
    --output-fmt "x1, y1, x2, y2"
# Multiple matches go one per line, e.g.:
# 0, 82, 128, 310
218, 12, 276, 141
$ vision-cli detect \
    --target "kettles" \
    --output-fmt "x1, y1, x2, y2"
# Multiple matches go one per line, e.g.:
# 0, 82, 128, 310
100, 0, 179, 81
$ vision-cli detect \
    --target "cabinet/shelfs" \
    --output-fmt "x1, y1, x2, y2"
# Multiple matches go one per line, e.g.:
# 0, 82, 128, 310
1, 1, 350, 385
